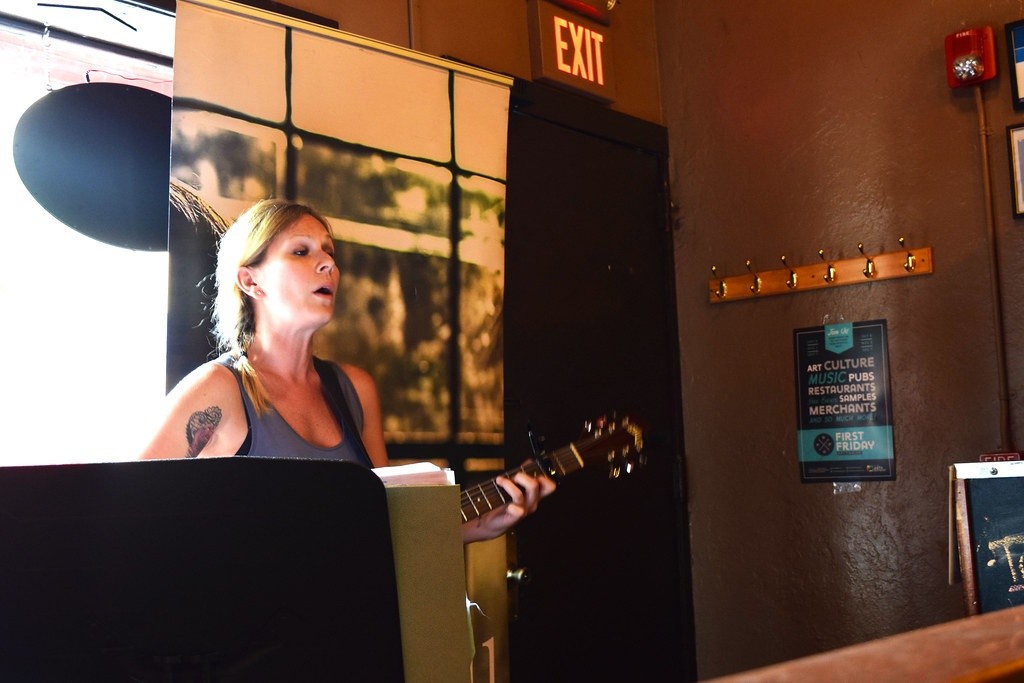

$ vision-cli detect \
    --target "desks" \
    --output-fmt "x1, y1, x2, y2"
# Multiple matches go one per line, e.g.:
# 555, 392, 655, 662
699, 605, 1024, 681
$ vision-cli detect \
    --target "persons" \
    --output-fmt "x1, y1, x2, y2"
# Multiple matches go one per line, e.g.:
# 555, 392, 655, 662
133, 199, 556, 545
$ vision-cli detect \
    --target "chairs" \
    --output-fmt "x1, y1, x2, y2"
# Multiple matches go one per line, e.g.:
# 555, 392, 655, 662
0, 458, 404, 682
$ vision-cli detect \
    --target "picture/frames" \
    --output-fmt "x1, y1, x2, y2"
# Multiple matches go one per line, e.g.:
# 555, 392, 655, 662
1004, 18, 1024, 113
1005, 122, 1024, 220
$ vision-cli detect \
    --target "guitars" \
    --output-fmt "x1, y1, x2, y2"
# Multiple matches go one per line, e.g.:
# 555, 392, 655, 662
460, 410, 648, 524
